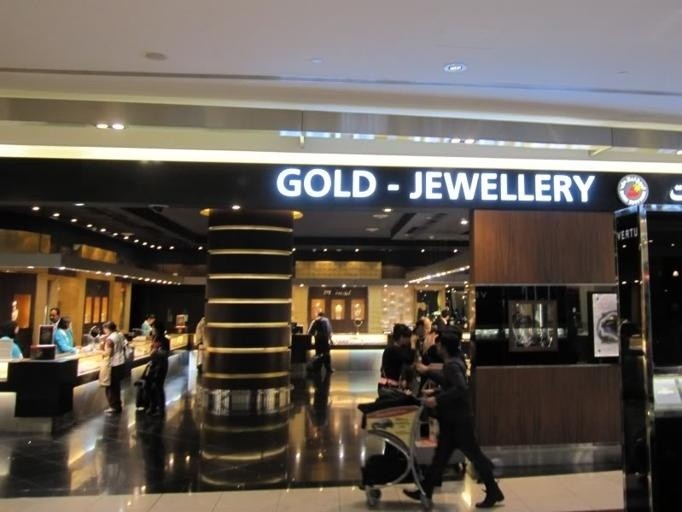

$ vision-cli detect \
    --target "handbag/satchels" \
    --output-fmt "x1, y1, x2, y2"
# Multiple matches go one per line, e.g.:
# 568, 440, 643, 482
135, 375, 164, 407
99, 356, 112, 387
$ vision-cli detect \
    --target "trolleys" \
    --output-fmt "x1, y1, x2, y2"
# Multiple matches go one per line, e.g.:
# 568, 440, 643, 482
356, 392, 435, 512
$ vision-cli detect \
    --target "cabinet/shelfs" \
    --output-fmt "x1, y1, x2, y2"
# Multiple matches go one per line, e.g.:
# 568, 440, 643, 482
1, 333, 202, 437
613, 203, 681, 511
307, 331, 392, 370
472, 208, 624, 482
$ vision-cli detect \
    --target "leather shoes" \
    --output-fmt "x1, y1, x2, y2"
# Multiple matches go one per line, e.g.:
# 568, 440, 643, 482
476, 490, 504, 507
403, 488, 433, 499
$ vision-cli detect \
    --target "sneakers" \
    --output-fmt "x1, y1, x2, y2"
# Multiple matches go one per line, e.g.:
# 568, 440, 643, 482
104, 407, 117, 412
135, 410, 165, 417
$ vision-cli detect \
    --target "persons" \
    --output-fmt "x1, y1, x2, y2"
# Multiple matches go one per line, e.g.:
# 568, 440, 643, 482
307, 311, 336, 373
0, 321, 23, 358
141, 313, 157, 335
146, 320, 170, 418
194, 317, 206, 344
378, 302, 505, 508
49, 307, 81, 353
102, 320, 129, 413
